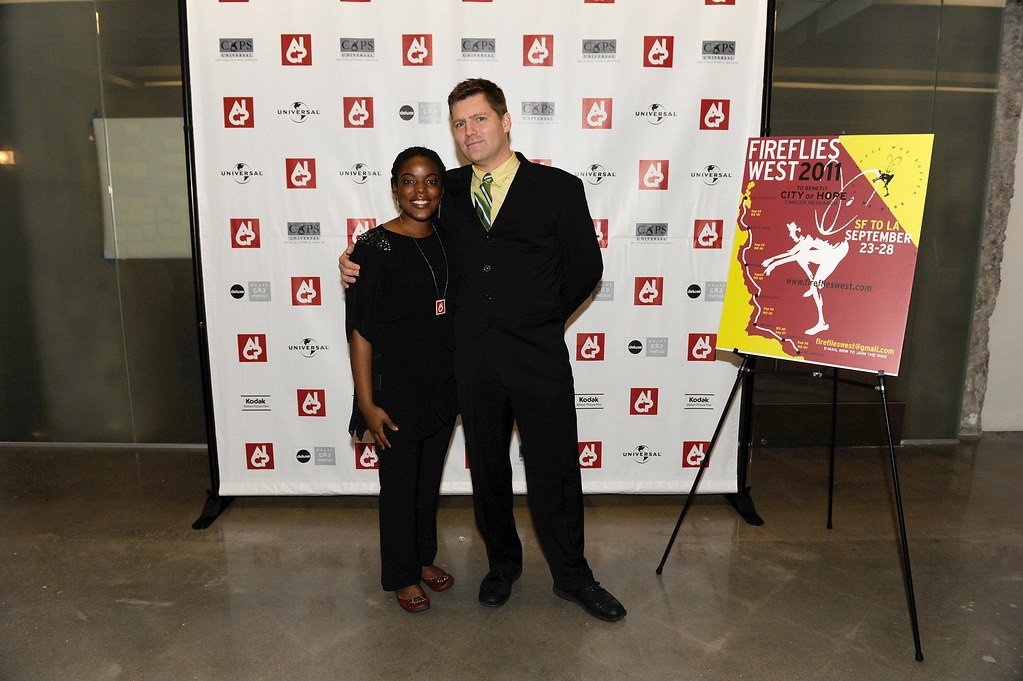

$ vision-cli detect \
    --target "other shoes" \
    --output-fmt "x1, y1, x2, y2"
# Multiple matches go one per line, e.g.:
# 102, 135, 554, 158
395, 587, 430, 613
422, 566, 454, 591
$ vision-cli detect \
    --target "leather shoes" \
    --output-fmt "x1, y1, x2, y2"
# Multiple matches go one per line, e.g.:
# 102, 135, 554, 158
479, 567, 522, 607
553, 580, 628, 622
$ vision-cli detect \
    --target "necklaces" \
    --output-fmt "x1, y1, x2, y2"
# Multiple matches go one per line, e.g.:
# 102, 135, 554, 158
400, 217, 448, 315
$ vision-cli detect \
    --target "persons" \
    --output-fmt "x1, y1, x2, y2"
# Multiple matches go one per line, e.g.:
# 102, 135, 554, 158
340, 79, 627, 622
348, 146, 460, 612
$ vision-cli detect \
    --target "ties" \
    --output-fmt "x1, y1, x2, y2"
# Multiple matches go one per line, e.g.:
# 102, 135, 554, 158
473, 173, 494, 231
